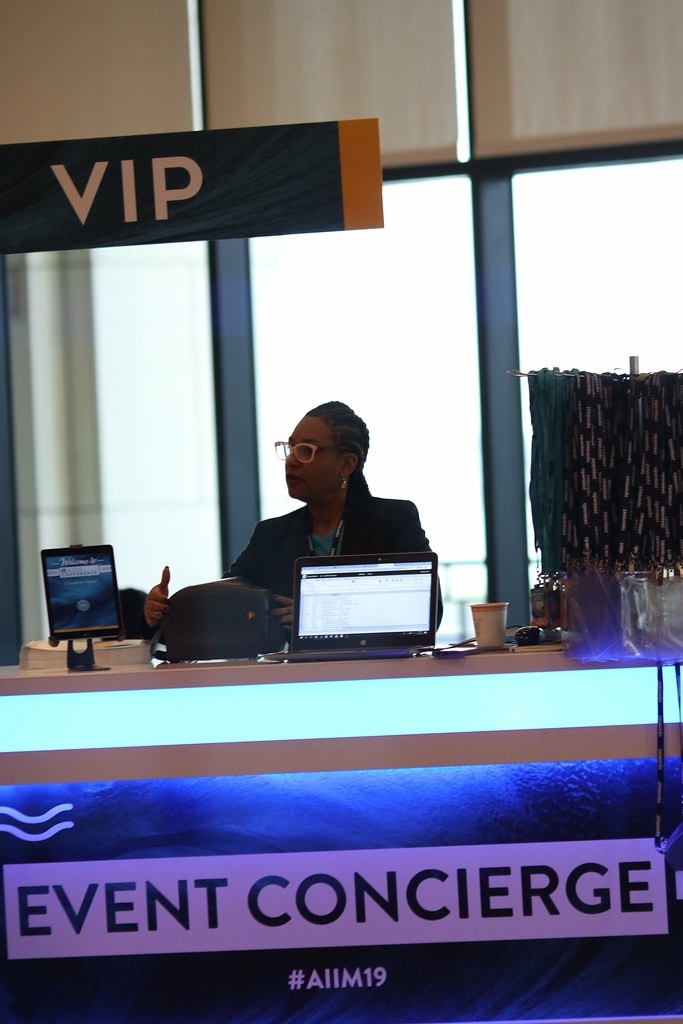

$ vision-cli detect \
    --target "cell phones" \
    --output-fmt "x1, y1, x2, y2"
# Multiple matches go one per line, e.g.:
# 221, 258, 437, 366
432, 645, 483, 658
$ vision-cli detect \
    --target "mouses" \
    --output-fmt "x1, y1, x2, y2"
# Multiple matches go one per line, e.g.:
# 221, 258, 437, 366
515, 626, 544, 645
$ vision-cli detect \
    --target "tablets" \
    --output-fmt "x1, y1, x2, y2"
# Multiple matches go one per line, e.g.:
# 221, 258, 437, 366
40, 545, 124, 640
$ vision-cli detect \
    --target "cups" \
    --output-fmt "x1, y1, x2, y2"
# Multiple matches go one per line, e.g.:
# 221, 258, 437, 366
469, 603, 509, 651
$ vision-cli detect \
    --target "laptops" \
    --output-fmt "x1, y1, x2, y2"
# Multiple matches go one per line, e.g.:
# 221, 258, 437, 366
264, 552, 438, 660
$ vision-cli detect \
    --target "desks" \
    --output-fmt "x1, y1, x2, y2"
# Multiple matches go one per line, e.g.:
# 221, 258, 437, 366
0, 650, 683, 1024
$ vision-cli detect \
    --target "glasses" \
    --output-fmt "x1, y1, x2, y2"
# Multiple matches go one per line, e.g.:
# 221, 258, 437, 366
275, 441, 353, 464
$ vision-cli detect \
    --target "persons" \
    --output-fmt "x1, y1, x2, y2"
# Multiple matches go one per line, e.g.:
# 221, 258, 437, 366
142, 400, 445, 654
